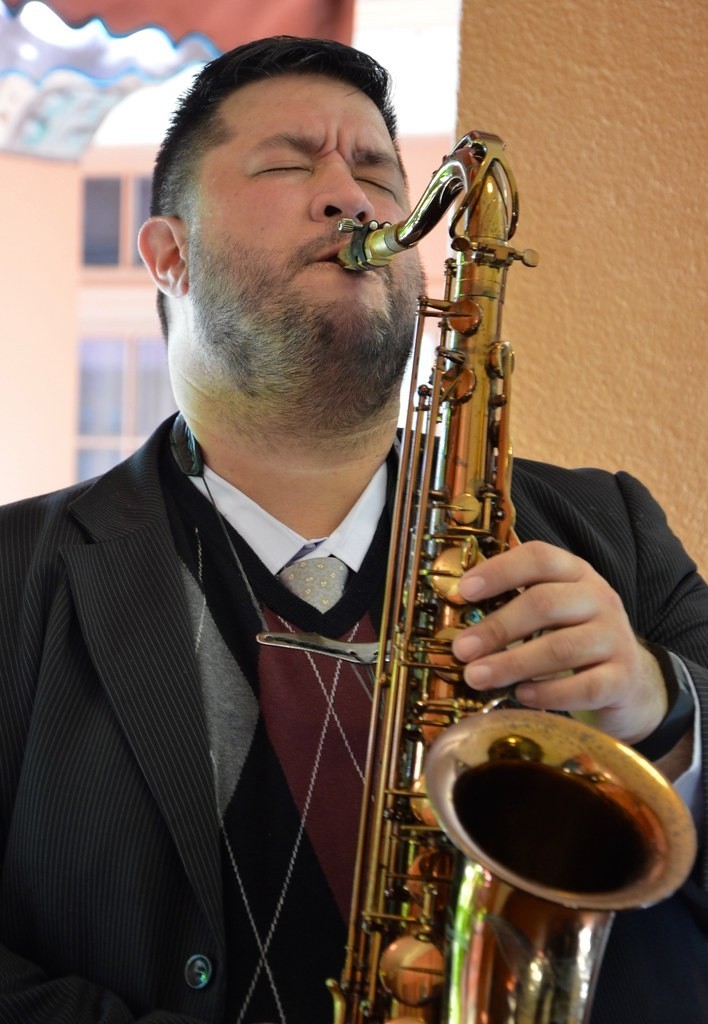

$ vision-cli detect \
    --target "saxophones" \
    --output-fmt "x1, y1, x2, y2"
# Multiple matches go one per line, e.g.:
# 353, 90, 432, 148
323, 129, 697, 1024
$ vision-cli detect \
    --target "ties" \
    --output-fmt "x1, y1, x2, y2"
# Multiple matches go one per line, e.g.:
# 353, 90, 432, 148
276, 556, 348, 615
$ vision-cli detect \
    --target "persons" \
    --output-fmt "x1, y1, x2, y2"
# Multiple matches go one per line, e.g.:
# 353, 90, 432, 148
2, 34, 708, 1024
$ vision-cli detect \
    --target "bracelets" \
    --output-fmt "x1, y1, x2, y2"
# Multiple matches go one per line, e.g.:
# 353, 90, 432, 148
627, 643, 696, 770
